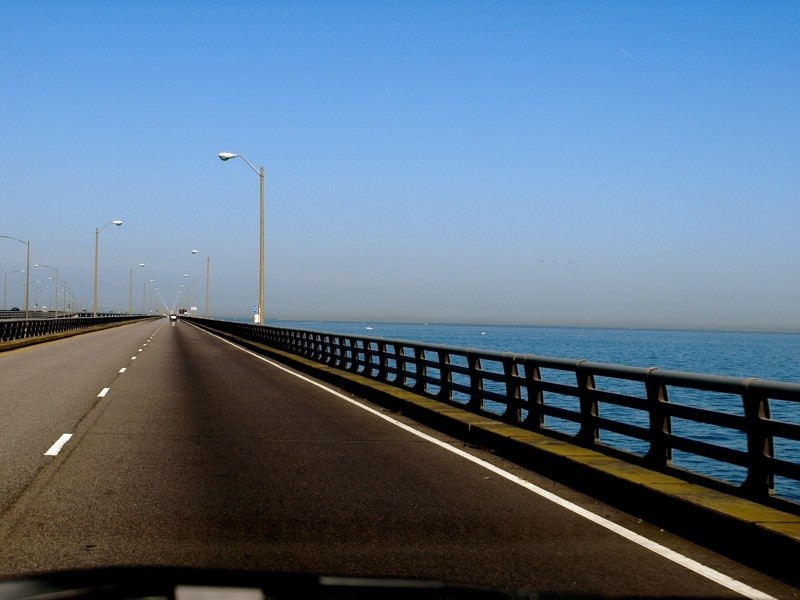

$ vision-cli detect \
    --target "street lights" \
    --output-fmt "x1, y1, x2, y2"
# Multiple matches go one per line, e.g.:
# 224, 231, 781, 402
191, 249, 209, 318
128, 263, 145, 316
31, 277, 74, 318
33, 264, 58, 318
3, 270, 25, 310
218, 152, 264, 326
94, 220, 124, 317
142, 274, 199, 316
